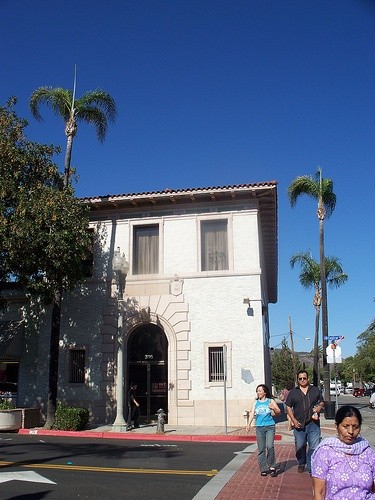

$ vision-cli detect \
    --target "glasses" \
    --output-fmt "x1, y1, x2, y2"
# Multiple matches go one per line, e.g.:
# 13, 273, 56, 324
298, 377, 308, 381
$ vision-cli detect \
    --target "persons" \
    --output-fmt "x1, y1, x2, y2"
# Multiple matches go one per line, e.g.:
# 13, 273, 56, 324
311, 405, 375, 500
286, 370, 325, 473
126, 383, 139, 428
247, 385, 281, 477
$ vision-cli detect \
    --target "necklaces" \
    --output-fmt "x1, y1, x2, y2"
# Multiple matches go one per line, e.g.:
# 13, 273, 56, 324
344, 452, 360, 472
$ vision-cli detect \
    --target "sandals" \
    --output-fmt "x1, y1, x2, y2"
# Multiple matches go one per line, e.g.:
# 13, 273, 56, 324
262, 470, 267, 476
270, 468, 276, 476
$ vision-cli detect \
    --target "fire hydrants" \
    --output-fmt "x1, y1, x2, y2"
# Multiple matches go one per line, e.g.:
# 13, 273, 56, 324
155, 409, 167, 434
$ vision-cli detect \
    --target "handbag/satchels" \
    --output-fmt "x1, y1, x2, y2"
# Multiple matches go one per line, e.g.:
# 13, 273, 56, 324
268, 399, 277, 416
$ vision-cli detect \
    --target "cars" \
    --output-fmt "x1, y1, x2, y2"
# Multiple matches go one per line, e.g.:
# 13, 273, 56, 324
328, 383, 375, 409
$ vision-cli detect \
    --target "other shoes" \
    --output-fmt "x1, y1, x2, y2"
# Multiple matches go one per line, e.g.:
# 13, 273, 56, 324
298, 461, 306, 473
